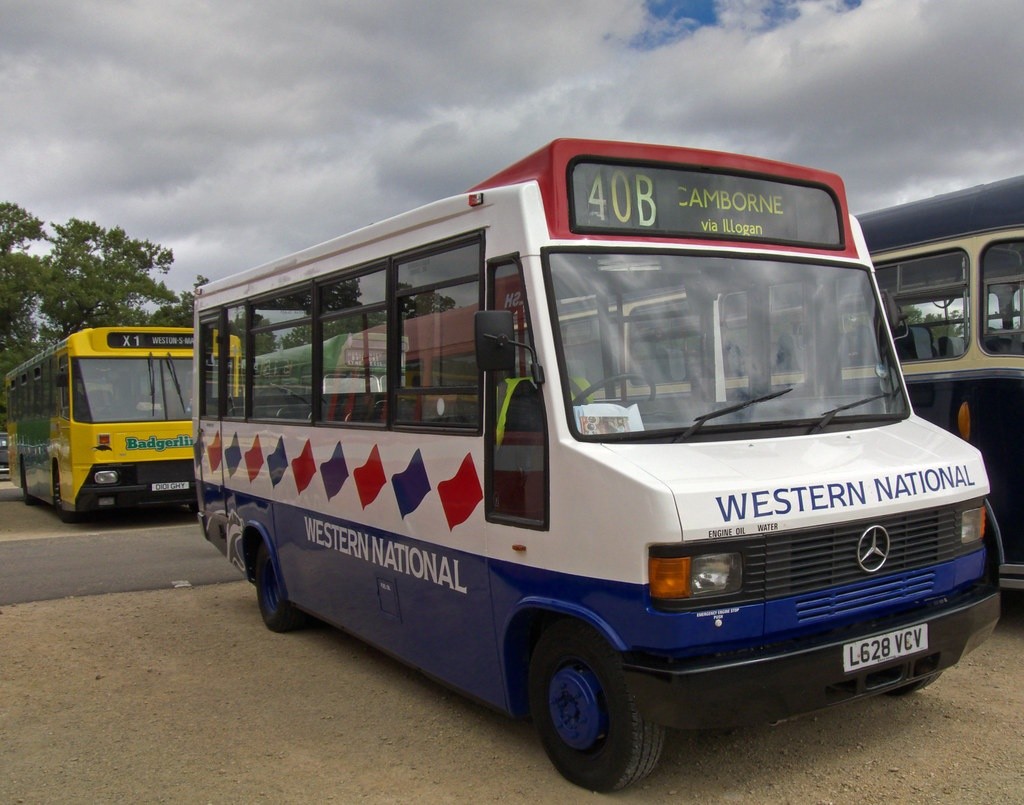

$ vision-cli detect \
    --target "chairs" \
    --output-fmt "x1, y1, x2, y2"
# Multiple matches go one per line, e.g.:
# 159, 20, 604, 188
938, 336, 965, 357
897, 326, 939, 361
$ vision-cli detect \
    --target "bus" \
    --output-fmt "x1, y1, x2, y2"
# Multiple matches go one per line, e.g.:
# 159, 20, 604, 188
512, 177, 1024, 591
230, 303, 479, 417
2, 325, 243, 524
188, 136, 1005, 792
